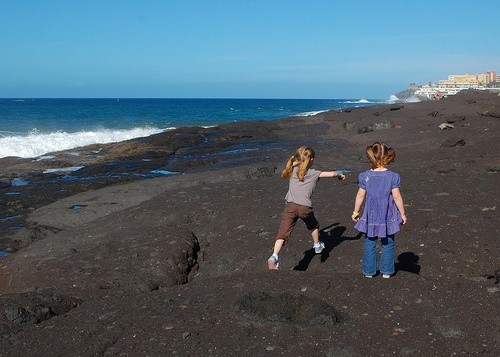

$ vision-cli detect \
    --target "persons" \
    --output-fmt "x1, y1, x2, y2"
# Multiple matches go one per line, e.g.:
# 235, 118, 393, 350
351, 142, 408, 278
267, 145, 346, 270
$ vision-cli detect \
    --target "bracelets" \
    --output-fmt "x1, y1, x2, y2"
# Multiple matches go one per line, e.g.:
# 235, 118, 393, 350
353, 210, 359, 216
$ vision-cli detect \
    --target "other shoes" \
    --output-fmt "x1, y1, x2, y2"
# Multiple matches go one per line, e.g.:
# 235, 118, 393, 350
365, 275, 372, 278
268, 255, 279, 270
313, 241, 325, 253
382, 274, 390, 279
